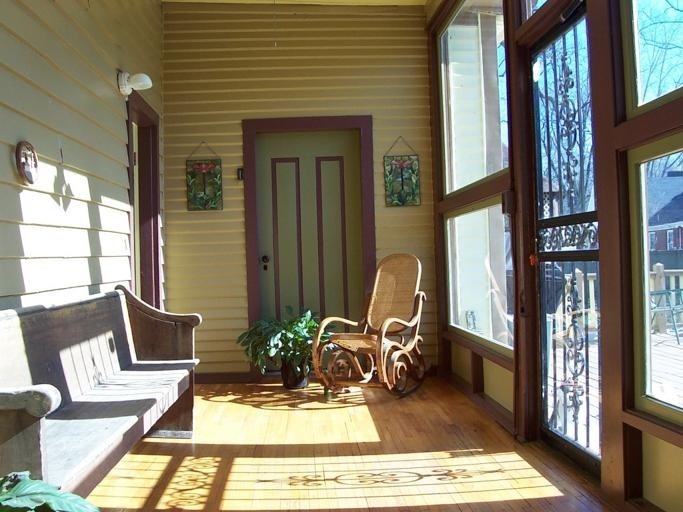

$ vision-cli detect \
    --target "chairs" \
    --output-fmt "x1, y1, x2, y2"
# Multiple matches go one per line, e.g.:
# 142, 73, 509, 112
312, 252, 433, 400
650, 288, 683, 347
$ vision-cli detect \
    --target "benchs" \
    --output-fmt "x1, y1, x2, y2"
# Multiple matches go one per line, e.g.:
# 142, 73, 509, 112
0, 284, 203, 512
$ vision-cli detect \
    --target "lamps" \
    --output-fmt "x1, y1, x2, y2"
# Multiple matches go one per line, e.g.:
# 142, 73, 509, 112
117, 69, 154, 97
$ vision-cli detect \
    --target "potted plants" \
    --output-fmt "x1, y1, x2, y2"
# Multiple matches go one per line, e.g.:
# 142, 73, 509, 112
235, 304, 337, 389
